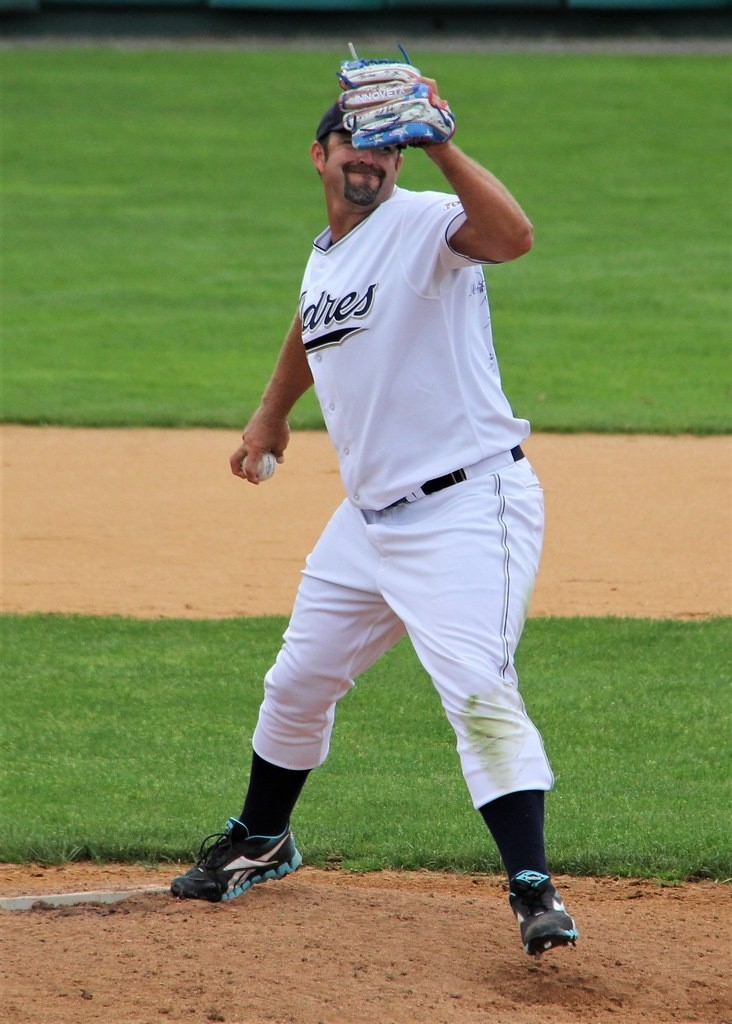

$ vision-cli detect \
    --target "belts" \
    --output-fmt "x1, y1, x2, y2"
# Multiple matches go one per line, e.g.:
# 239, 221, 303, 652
390, 444, 524, 506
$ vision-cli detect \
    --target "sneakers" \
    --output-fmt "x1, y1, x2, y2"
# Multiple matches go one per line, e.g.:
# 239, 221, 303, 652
509, 869, 579, 953
171, 816, 303, 903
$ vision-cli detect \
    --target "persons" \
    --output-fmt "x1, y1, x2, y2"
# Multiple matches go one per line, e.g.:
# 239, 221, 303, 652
170, 59, 578, 957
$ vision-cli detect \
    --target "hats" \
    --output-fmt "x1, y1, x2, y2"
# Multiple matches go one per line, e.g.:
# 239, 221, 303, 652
316, 102, 346, 141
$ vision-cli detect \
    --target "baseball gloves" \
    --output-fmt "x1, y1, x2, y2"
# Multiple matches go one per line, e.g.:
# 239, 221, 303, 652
334, 39, 457, 152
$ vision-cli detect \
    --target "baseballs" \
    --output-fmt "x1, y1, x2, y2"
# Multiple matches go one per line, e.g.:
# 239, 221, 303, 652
241, 451, 277, 481
